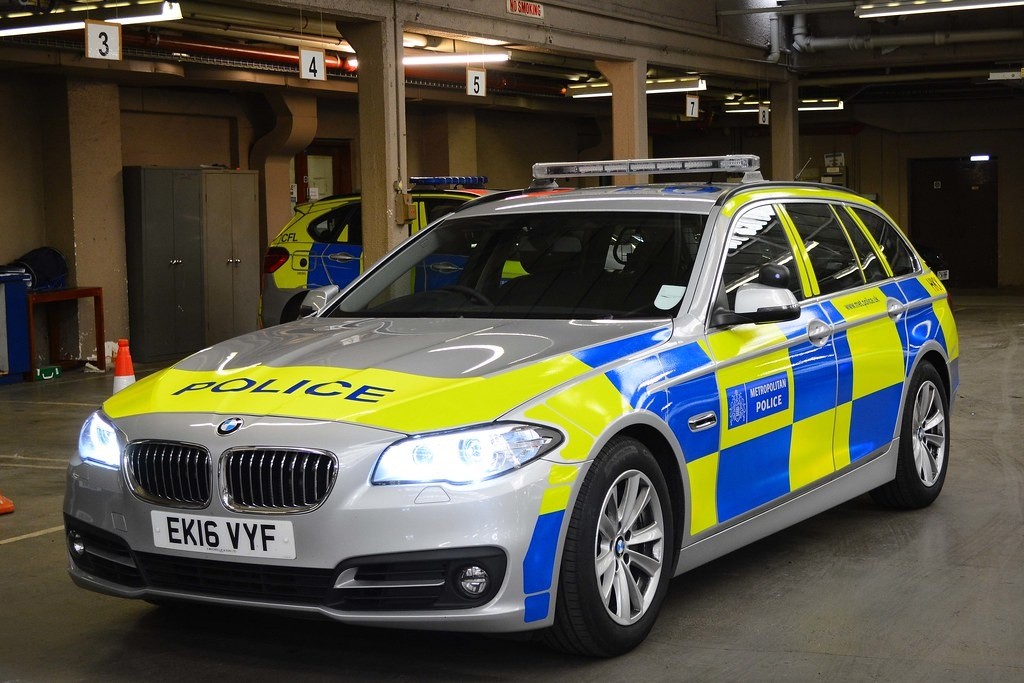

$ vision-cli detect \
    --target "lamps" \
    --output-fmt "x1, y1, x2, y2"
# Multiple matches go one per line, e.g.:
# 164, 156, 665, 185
722, 96, 844, 113
0, 0, 184, 36
854, 0, 1024, 18
565, 75, 707, 98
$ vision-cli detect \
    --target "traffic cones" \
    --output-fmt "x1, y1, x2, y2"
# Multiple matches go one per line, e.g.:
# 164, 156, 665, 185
112, 338, 136, 394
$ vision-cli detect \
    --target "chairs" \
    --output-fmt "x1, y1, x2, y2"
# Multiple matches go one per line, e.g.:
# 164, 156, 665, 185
610, 221, 729, 318
493, 220, 620, 319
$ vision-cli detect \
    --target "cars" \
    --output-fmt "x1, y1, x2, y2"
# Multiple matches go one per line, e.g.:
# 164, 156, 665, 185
259, 175, 534, 327
62, 155, 963, 658
